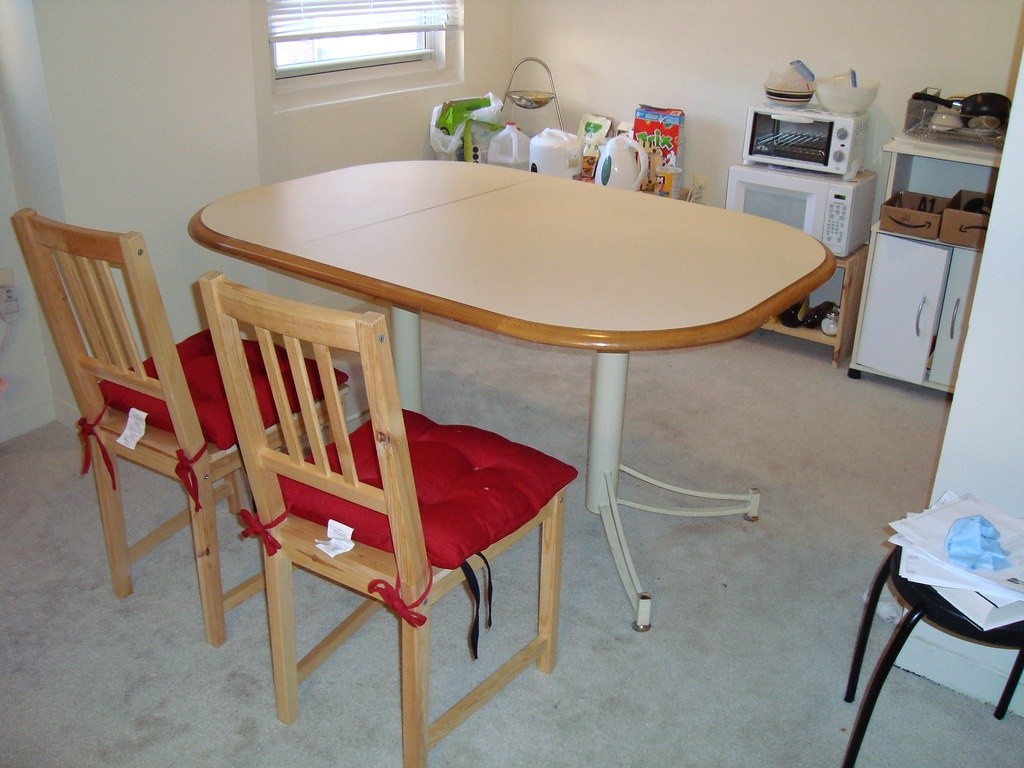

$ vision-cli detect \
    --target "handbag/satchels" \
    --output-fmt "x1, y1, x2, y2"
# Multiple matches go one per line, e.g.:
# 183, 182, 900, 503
429, 91, 504, 160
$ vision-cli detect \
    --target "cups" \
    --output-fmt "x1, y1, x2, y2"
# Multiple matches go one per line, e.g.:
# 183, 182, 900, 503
655, 167, 682, 200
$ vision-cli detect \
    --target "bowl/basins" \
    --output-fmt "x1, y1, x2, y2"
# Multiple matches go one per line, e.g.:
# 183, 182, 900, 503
762, 82, 814, 106
928, 105, 963, 131
812, 74, 880, 117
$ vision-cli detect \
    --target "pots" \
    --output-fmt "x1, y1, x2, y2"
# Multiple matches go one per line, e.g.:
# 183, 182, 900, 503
911, 89, 1011, 131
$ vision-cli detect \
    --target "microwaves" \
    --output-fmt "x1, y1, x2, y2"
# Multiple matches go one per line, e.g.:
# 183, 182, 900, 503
725, 164, 878, 258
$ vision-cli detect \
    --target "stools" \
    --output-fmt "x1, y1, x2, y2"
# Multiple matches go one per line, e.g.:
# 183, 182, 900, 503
839, 544, 1024, 768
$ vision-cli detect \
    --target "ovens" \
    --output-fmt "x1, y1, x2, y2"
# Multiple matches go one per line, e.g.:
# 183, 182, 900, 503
741, 103, 870, 180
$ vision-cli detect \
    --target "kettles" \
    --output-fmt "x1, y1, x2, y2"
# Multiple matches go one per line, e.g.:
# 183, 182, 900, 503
595, 135, 648, 192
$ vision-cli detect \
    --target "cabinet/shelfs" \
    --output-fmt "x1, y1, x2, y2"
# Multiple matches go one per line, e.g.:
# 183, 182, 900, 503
846, 220, 985, 393
761, 244, 868, 370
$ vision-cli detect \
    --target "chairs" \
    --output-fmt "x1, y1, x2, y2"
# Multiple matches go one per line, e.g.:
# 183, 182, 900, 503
10, 208, 351, 648
193, 265, 580, 767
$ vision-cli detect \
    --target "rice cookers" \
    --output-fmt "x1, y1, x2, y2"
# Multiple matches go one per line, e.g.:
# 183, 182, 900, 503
529, 128, 582, 183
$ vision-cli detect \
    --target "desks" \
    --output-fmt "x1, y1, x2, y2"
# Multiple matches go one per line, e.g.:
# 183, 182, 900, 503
187, 160, 836, 630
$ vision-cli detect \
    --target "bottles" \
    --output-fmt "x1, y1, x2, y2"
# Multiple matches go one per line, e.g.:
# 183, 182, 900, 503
821, 311, 838, 336
487, 121, 531, 170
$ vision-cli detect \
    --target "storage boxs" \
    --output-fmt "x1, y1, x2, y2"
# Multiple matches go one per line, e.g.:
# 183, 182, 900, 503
879, 189, 952, 241
900, 544, 1024, 633
433, 94, 522, 167
939, 190, 994, 250
631, 103, 685, 168
